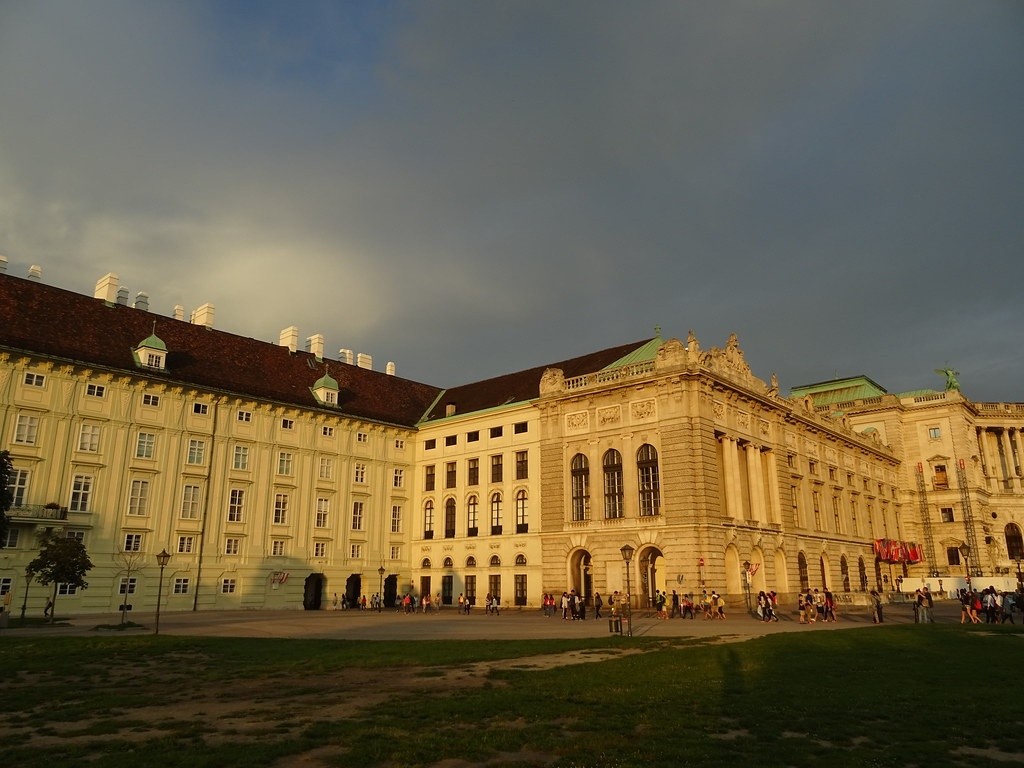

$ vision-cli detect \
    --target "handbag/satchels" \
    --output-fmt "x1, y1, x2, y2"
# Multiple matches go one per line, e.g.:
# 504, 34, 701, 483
705, 594, 710, 603
994, 603, 999, 609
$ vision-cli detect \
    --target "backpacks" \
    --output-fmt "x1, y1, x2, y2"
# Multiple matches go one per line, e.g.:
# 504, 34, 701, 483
961, 605, 967, 611
719, 598, 725, 606
657, 594, 665, 603
608, 595, 614, 606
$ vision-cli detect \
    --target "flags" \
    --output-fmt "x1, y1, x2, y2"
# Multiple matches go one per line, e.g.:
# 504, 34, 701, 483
749, 564, 760, 576
874, 538, 925, 565
278, 572, 289, 584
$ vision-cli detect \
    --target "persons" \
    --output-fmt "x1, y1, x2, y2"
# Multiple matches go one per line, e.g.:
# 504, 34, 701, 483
332, 592, 380, 611
996, 536, 1005, 558
396, 593, 442, 616
945, 366, 960, 388
913, 586, 935, 624
44, 597, 52, 617
798, 587, 837, 625
870, 590, 882, 623
485, 593, 500, 617
457, 593, 471, 615
757, 591, 779, 623
687, 329, 699, 359
956, 586, 1016, 625
543, 589, 618, 622
656, 589, 727, 621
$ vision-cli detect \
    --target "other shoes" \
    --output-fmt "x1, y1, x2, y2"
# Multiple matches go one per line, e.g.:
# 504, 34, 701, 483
711, 619, 712, 621
767, 620, 772, 624
594, 619, 598, 621
720, 619, 723, 620
683, 616, 685, 619
497, 614, 499, 616
485, 614, 487, 617
408, 612, 410, 615
490, 613, 494, 616
803, 623, 806, 624
362, 610, 363, 613
978, 622, 983, 624
760, 621, 766, 623
690, 618, 693, 619
600, 618, 602, 621
775, 619, 779, 622
703, 619, 706, 621
464, 612, 466, 615
808, 621, 812, 624
716, 618, 718, 620
413, 612, 414, 615
871, 621, 874, 624
724, 617, 727, 620
670, 617, 673, 619
810, 618, 816, 622
829, 619, 834, 622
800, 623, 802, 624
974, 622, 977, 624
821, 619, 828, 622
960, 621, 963, 624
458, 611, 461, 615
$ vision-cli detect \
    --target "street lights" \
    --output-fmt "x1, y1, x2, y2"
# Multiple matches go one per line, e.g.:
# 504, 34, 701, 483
743, 561, 756, 611
156, 548, 172, 633
1014, 551, 1024, 589
620, 544, 634, 637
377, 565, 386, 613
958, 541, 973, 594
